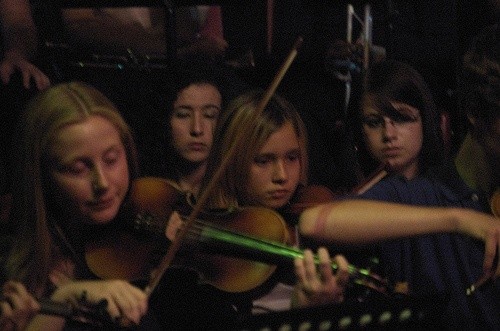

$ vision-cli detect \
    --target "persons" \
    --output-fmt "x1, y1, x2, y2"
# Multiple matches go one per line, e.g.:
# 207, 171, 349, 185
0, 0, 500, 331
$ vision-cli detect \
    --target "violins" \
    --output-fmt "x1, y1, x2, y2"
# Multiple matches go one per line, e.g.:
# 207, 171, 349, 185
286, 180, 345, 225
85, 176, 414, 305
